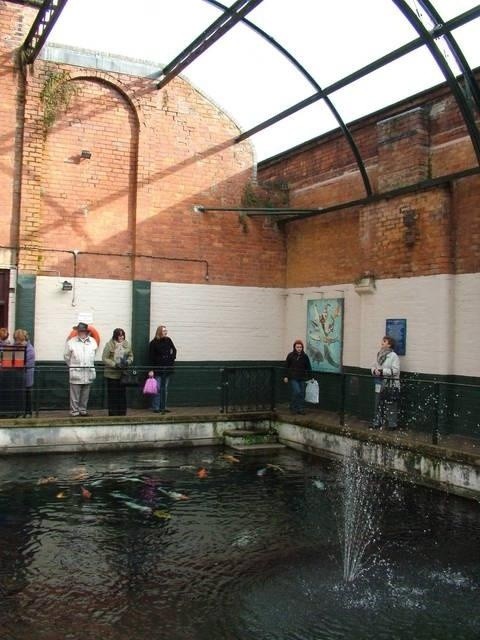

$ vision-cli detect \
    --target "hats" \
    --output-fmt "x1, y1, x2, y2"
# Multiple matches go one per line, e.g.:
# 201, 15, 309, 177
73, 323, 91, 332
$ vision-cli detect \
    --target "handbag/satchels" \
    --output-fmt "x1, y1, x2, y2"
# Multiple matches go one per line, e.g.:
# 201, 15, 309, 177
120, 369, 143, 388
380, 386, 401, 402
143, 376, 157, 395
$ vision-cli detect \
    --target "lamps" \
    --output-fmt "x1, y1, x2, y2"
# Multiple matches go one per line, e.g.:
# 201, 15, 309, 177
80, 150, 92, 159
62, 281, 72, 291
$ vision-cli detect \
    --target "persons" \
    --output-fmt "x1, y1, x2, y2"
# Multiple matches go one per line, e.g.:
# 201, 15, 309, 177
64, 322, 98, 417
282, 341, 315, 415
145, 325, 177, 413
101, 328, 134, 416
369, 336, 401, 431
0, 328, 35, 419
